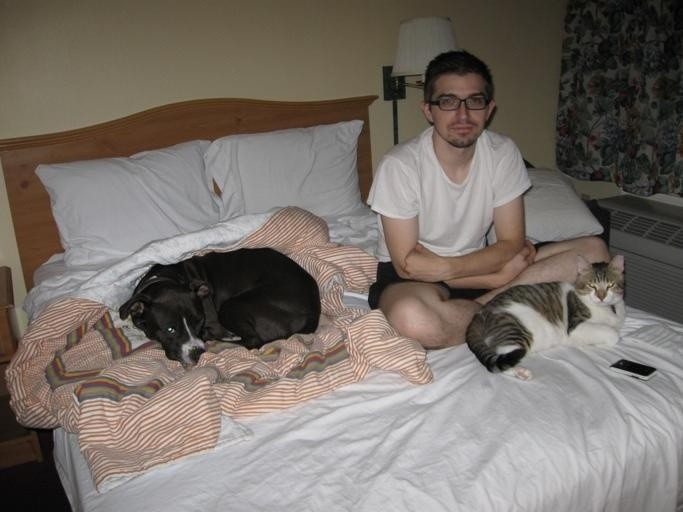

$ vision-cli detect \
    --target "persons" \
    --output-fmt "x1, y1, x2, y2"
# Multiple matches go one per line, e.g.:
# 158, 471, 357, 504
366, 50, 625, 352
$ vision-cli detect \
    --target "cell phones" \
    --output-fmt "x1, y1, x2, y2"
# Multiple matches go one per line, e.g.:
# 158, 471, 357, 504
609, 359, 657, 380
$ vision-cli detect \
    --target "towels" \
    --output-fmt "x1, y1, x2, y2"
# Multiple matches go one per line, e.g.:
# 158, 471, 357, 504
616, 320, 683, 370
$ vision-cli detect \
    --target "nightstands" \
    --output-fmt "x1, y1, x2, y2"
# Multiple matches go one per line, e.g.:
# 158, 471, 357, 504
0, 267, 44, 472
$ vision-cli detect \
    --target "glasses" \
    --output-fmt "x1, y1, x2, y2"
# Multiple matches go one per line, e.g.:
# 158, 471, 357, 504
430, 95, 489, 110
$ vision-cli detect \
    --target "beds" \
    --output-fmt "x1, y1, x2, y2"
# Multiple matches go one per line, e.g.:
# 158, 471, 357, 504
1, 95, 683, 512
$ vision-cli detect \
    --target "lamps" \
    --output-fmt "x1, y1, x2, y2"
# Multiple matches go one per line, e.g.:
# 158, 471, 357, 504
390, 17, 459, 91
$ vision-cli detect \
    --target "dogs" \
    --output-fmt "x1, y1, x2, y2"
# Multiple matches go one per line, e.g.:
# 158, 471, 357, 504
117, 247, 322, 370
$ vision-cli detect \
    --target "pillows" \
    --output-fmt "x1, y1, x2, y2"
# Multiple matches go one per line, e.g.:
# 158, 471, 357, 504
202, 119, 369, 224
35, 139, 222, 271
485, 168, 604, 245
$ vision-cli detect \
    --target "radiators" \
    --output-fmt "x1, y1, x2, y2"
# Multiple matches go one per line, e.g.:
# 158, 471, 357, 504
585, 195, 683, 325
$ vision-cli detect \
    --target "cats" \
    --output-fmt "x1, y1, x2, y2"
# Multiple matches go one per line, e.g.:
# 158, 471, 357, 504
464, 253, 625, 381
